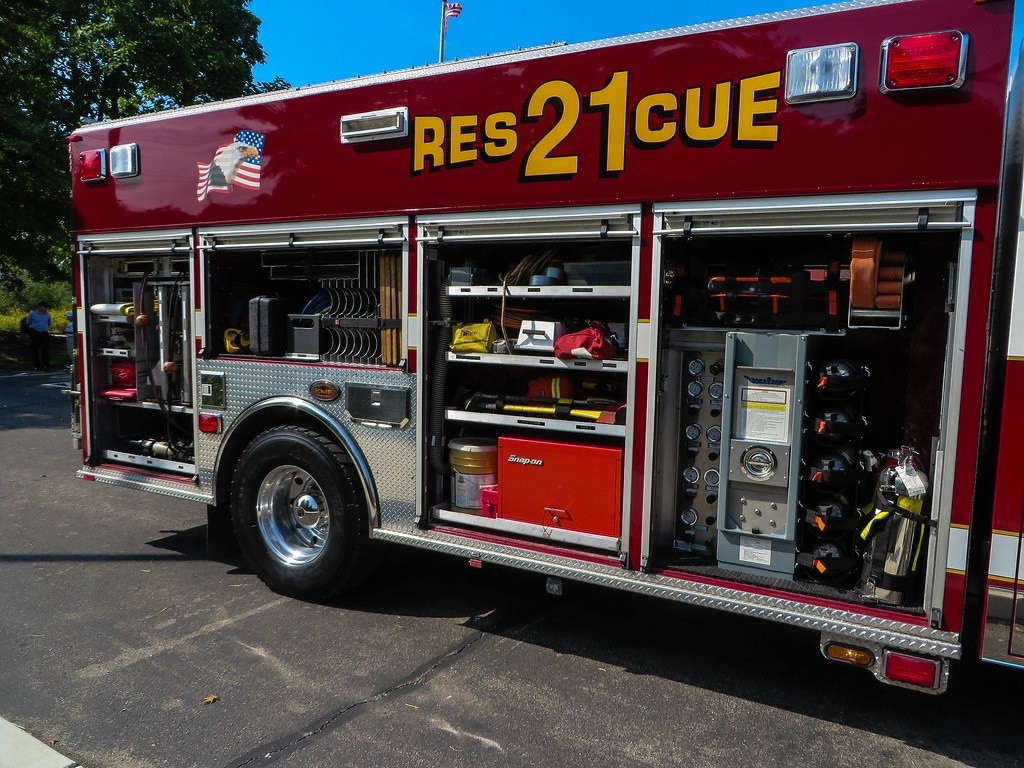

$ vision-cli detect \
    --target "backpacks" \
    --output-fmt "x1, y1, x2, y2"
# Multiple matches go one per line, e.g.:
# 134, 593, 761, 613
19, 317, 30, 335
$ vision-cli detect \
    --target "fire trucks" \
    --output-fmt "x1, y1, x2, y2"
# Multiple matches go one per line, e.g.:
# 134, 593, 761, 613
64, 0, 1024, 695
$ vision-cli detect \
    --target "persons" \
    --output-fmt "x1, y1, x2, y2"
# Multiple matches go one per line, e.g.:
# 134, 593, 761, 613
26, 302, 51, 371
62, 313, 76, 369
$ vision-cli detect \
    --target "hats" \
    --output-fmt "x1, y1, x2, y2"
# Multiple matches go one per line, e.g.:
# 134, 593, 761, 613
64, 312, 73, 316
39, 301, 50, 307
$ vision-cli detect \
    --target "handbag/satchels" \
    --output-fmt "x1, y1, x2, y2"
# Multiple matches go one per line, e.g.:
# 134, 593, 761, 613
554, 320, 619, 359
448, 319, 497, 354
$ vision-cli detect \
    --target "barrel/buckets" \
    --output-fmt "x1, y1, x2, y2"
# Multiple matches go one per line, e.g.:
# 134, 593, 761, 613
448, 437, 498, 510
248, 295, 287, 356
